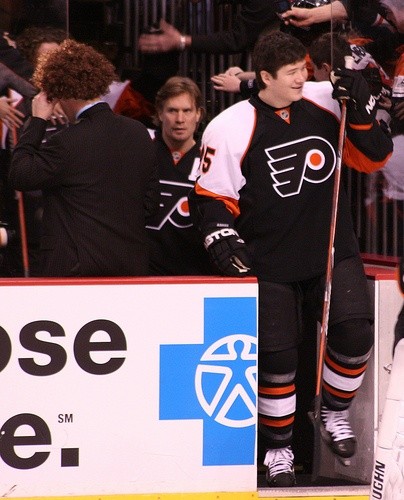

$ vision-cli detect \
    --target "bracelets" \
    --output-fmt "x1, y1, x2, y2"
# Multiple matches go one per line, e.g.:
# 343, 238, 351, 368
178, 35, 186, 50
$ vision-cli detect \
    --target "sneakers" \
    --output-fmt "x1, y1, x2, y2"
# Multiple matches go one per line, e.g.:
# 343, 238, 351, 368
320, 403, 357, 458
263, 444, 296, 487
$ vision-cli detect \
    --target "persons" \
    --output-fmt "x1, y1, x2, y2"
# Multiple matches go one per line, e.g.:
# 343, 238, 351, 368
0, 0, 404, 486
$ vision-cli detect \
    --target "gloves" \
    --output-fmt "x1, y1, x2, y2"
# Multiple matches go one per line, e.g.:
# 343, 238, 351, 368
330, 67, 377, 123
199, 222, 252, 277
